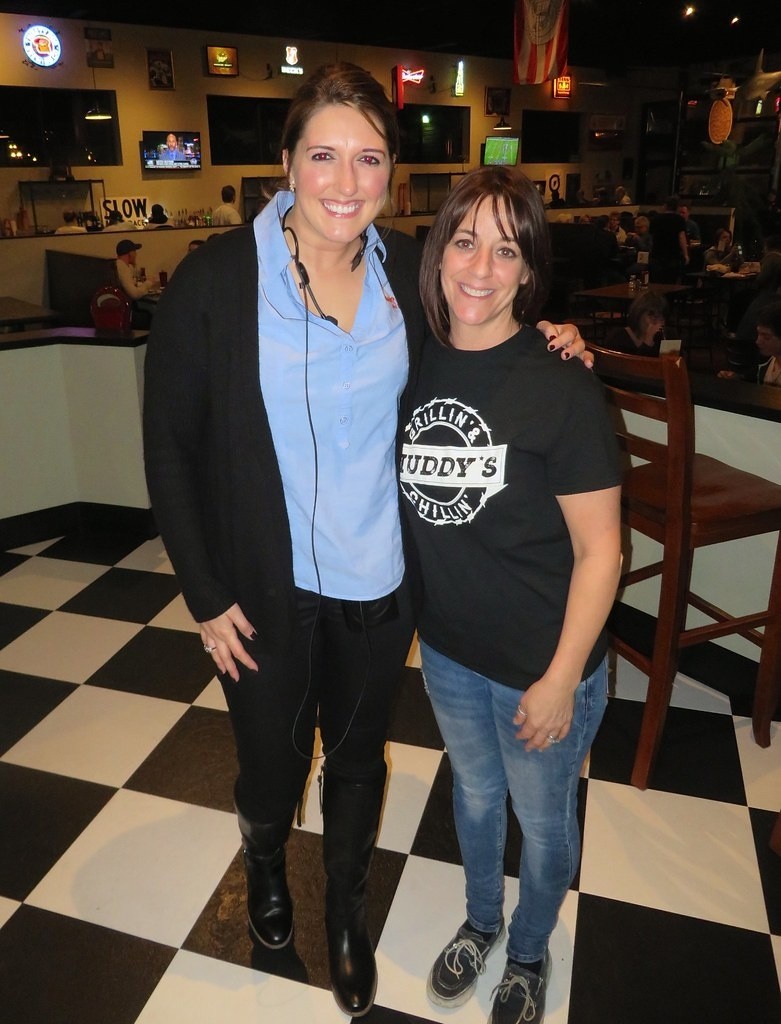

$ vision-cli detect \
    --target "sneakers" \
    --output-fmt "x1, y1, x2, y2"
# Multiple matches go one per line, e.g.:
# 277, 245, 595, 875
426, 917, 506, 1008
489, 951, 552, 1024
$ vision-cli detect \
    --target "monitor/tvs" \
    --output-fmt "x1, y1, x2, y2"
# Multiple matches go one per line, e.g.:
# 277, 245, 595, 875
142, 130, 201, 171
483, 136, 520, 166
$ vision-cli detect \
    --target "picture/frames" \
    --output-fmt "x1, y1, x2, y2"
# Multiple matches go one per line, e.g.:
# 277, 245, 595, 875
484, 87, 513, 118
145, 48, 175, 90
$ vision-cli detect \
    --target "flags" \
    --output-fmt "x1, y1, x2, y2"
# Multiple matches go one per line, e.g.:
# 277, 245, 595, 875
512, 1, 570, 85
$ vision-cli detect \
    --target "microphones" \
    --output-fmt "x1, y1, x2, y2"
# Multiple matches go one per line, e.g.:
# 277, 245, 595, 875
306, 284, 338, 326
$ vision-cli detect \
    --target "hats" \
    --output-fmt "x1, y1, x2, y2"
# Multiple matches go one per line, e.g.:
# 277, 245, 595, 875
116, 241, 142, 256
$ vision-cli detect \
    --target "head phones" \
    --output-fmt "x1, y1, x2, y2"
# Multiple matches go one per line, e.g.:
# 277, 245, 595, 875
281, 205, 368, 285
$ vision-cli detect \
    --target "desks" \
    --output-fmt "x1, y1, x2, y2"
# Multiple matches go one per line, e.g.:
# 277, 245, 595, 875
706, 272, 758, 326
574, 282, 691, 342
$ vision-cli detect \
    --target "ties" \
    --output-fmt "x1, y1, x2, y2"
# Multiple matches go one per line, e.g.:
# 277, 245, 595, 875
171, 153, 174, 159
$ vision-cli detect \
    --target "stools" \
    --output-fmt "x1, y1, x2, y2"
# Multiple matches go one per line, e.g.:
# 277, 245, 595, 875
558, 344, 781, 791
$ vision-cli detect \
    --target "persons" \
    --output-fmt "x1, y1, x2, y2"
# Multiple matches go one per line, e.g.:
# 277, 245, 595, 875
575, 201, 712, 305
704, 227, 754, 339
720, 120, 775, 170
394, 162, 627, 1024
716, 308, 781, 386
148, 132, 187, 161
114, 239, 157, 331
103, 210, 138, 233
610, 287, 690, 399
729, 254, 781, 383
249, 199, 266, 224
144, 58, 594, 1017
54, 208, 88, 234
94, 41, 108, 61
212, 185, 242, 226
143, 204, 176, 230
599, 141, 623, 191
760, 188, 781, 244
187, 239, 206, 255
615, 186, 632, 205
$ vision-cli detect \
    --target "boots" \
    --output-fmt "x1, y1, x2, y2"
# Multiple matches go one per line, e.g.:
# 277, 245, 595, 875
317, 759, 387, 1017
232, 775, 297, 951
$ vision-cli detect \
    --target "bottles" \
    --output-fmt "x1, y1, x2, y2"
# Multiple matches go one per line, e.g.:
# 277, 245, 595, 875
628, 274, 642, 291
404, 200, 411, 216
70, 210, 101, 232
164, 206, 213, 227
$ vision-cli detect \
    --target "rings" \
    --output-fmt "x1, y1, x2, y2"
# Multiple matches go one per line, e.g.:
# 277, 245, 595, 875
547, 735, 561, 745
202, 644, 216, 653
518, 705, 526, 716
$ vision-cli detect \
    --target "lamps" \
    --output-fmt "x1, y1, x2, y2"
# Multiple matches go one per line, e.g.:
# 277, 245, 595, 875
84, 0, 113, 120
493, 116, 512, 130
